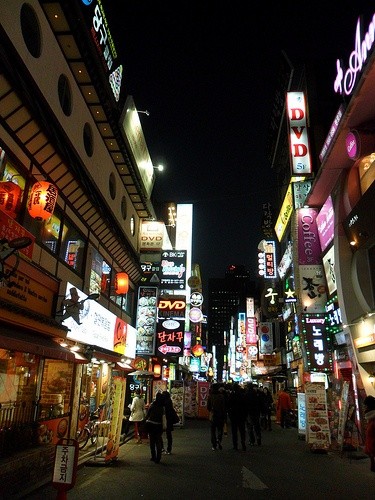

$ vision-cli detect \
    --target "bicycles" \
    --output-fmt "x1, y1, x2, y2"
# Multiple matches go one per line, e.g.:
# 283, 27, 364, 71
78, 407, 100, 450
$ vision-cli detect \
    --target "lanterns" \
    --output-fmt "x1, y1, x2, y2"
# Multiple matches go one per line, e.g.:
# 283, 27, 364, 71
114, 272, 128, 294
101, 273, 106, 293
0, 181, 22, 218
27, 181, 57, 222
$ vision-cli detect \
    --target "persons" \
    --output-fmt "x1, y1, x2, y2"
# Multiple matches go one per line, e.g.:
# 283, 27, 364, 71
277, 388, 291, 430
145, 391, 180, 464
364, 395, 375, 472
128, 388, 145, 441
207, 382, 273, 450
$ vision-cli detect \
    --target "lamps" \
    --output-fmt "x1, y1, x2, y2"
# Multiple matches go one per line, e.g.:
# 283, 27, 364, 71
51, 293, 100, 319
0, 236, 32, 263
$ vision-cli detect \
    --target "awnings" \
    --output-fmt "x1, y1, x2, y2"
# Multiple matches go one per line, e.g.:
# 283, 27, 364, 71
252, 368, 287, 379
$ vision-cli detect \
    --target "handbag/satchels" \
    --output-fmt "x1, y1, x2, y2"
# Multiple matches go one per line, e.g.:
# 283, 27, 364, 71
172, 411, 180, 425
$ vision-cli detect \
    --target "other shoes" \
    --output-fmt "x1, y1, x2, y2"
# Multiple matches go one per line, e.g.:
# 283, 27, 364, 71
162, 449, 165, 452
212, 447, 215, 451
216, 439, 222, 450
165, 451, 171, 454
232, 446, 236, 450
135, 438, 142, 444
150, 458, 154, 461
120, 436, 126, 443
248, 441, 254, 446
